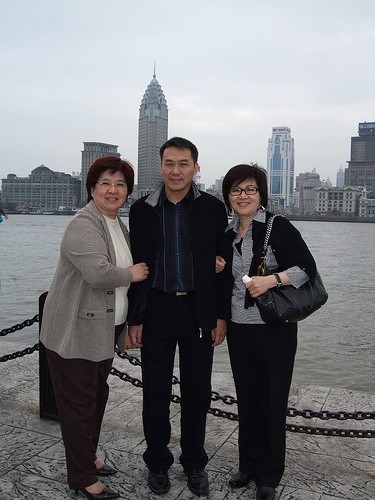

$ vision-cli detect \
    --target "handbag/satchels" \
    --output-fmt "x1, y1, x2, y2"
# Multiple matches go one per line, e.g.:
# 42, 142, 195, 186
256, 213, 330, 325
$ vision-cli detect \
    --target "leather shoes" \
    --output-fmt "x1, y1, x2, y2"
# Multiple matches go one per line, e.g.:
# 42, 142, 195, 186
147, 466, 170, 495
228, 470, 254, 489
182, 465, 210, 497
256, 486, 276, 500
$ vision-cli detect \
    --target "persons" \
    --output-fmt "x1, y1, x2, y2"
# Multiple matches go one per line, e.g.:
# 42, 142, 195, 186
128, 138, 231, 498
37, 156, 148, 500
214, 163, 316, 500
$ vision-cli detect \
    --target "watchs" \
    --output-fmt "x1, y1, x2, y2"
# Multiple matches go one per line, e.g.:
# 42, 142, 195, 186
274, 273, 282, 287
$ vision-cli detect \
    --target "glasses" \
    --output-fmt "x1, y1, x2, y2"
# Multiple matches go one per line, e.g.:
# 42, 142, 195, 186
229, 185, 259, 196
97, 178, 128, 190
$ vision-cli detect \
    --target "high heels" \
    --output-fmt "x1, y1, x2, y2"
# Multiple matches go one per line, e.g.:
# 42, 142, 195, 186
73, 483, 121, 500
96, 463, 119, 476
